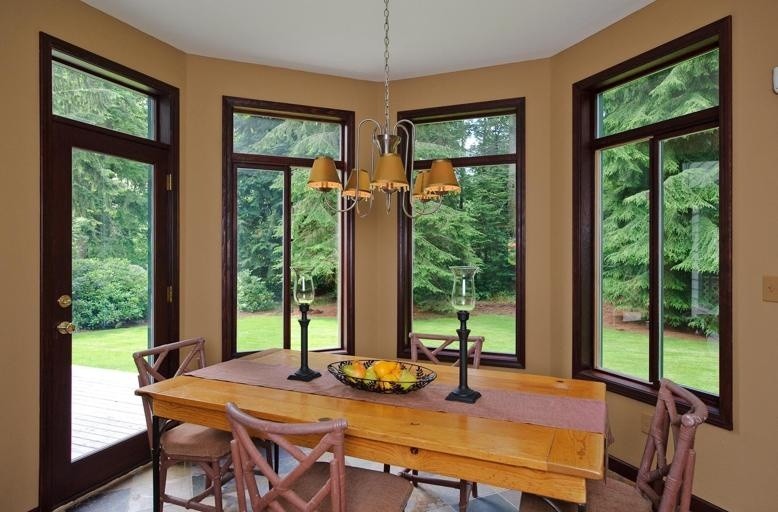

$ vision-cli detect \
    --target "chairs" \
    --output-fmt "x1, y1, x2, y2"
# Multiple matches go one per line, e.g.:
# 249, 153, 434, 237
226, 399, 414, 512
518, 377, 709, 512
132, 336, 273, 512
399, 332, 485, 512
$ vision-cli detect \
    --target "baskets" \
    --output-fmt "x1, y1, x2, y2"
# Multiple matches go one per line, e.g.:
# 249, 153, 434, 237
328, 360, 438, 395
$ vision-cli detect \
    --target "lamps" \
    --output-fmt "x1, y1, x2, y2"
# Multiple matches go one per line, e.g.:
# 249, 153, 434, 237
305, 0, 462, 218
445, 265, 482, 404
287, 265, 322, 382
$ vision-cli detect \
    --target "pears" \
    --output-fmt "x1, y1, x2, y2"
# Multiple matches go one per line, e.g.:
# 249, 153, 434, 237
342, 360, 417, 392
